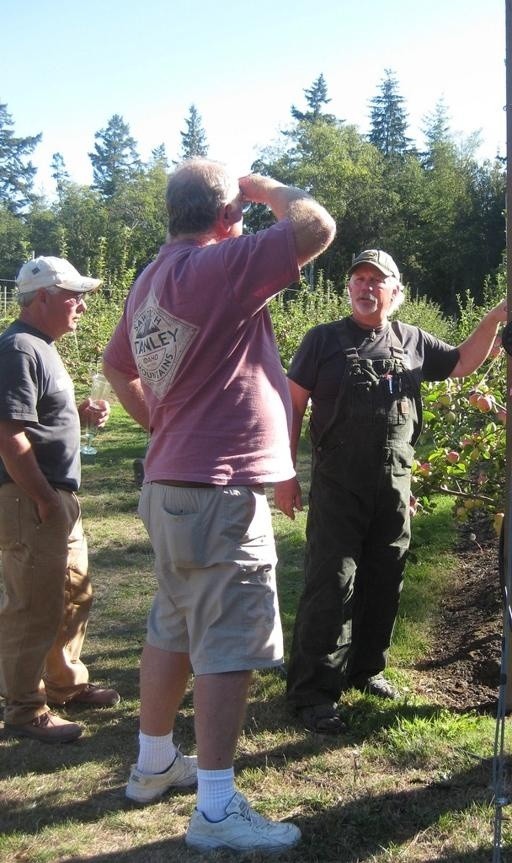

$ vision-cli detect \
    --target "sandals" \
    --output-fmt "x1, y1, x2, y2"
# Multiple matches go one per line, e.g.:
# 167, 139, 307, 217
365, 678, 395, 699
298, 704, 346, 735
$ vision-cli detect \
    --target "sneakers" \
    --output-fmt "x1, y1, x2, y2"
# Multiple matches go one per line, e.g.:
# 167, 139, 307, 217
48, 684, 120, 705
7, 709, 83, 742
126, 744, 198, 802
186, 792, 301, 852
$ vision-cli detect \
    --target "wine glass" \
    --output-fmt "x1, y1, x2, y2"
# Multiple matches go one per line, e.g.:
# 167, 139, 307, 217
80, 375, 113, 457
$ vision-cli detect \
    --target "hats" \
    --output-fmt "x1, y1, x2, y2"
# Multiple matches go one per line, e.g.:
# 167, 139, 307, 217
348, 249, 400, 279
17, 256, 103, 294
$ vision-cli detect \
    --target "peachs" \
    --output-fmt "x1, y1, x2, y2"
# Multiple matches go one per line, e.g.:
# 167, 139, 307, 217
409, 337, 506, 536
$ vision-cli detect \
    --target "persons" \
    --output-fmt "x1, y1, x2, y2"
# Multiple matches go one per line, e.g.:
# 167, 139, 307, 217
273, 250, 508, 734
0, 254, 121, 744
103, 158, 337, 853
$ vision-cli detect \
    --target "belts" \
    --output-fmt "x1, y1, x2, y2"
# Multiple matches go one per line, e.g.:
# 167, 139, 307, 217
151, 479, 212, 488
